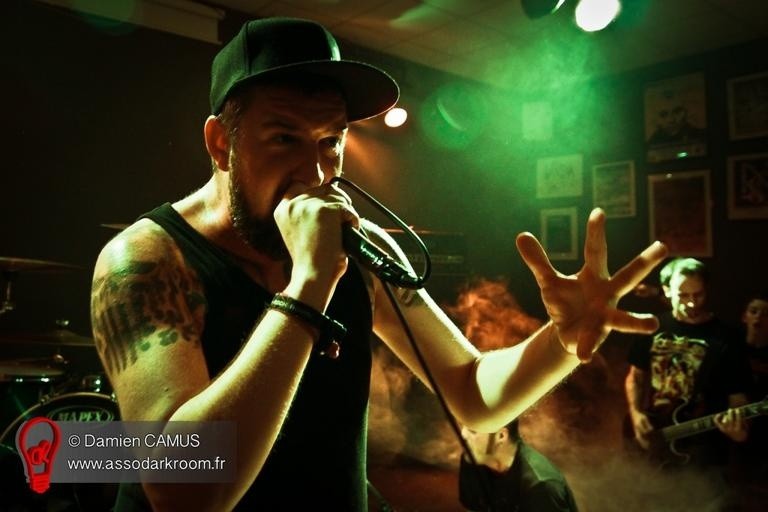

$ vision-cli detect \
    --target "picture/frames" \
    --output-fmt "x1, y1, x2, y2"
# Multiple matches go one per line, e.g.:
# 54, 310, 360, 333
539, 206, 579, 262
646, 166, 715, 263
725, 70, 766, 140
725, 148, 767, 222
590, 160, 637, 220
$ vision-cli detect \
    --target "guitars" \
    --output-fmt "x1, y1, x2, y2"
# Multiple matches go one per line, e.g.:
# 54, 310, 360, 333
621, 395, 767, 479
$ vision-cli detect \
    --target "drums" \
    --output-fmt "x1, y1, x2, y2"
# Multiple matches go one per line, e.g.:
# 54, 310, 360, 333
1, 390, 120, 511
0, 354, 117, 442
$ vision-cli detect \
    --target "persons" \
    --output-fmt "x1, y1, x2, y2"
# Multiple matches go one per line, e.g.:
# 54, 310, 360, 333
89, 16, 669, 512
648, 88, 704, 144
622, 255, 753, 469
739, 294, 768, 401
458, 416, 579, 512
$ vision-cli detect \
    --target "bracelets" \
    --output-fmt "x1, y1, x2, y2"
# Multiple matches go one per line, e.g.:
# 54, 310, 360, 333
270, 289, 347, 361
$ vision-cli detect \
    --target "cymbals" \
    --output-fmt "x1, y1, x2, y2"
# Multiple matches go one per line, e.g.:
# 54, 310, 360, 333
0, 256, 85, 277
98, 222, 133, 230
1, 324, 95, 348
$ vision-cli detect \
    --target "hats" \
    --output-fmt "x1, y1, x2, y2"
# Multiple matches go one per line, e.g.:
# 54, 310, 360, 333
208, 15, 401, 124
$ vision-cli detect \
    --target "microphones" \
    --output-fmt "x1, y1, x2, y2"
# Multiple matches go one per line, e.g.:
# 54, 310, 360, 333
336, 219, 421, 293
